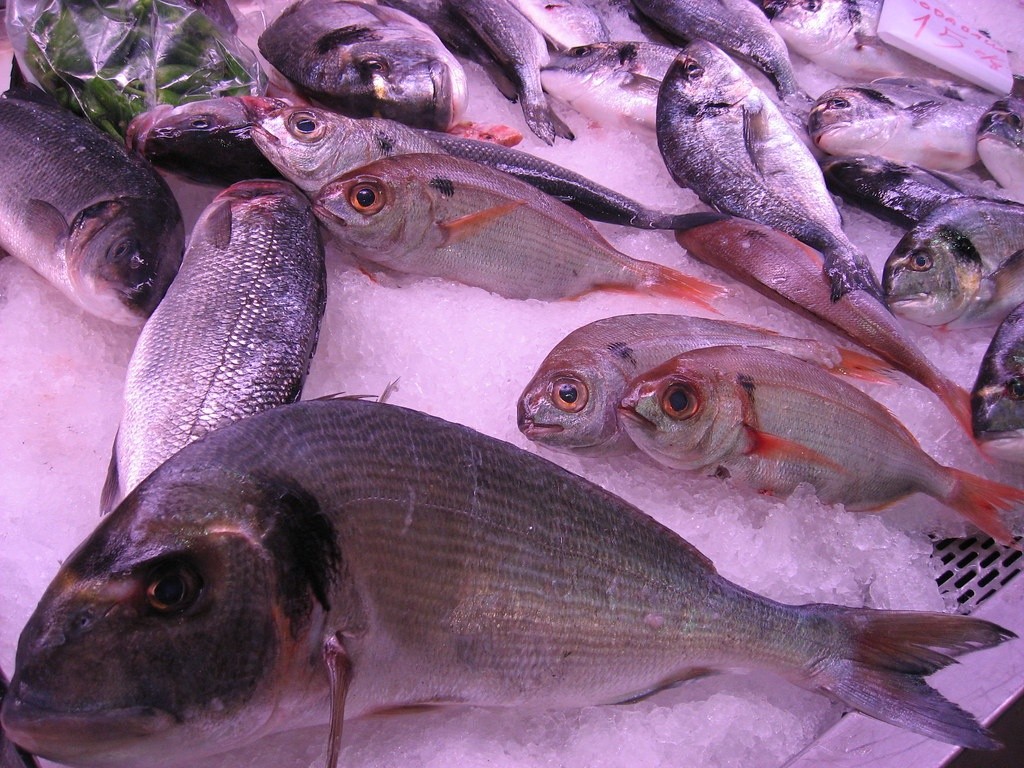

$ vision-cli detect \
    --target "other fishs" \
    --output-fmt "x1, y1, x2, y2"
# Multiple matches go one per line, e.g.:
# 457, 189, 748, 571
0, 0, 1024, 768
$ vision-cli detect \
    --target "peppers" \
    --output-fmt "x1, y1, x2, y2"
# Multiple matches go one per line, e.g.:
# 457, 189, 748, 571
20, 0, 269, 146
